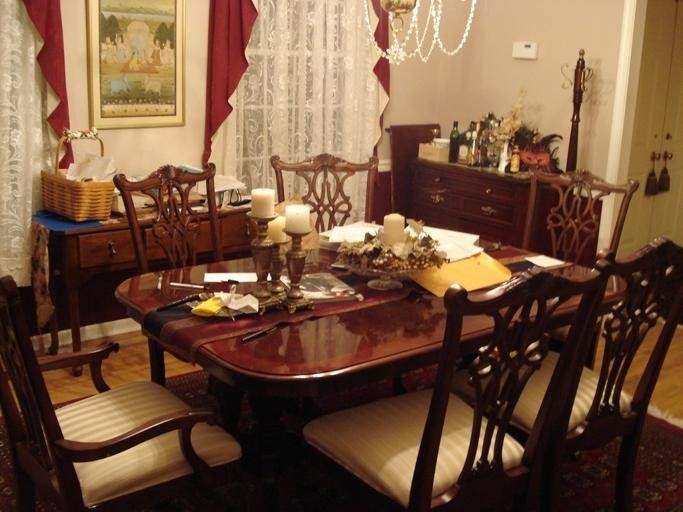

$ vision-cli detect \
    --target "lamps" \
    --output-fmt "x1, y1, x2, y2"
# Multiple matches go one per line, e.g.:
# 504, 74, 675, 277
379, 0, 417, 33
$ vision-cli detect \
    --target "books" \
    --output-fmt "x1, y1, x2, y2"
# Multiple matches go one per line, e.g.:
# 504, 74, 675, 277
278, 269, 365, 305
112, 163, 247, 217
317, 219, 485, 263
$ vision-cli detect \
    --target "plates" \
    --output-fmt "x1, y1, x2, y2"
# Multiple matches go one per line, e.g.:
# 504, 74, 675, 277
188, 300, 243, 318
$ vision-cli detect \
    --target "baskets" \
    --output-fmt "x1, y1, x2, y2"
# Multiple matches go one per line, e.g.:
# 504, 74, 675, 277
41, 128, 115, 221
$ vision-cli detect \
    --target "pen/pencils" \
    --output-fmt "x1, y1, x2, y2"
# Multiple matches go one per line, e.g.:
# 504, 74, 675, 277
170, 281, 209, 291
241, 321, 281, 342
156, 275, 163, 291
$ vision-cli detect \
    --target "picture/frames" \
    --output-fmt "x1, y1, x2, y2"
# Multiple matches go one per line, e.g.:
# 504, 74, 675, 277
87, 1, 186, 130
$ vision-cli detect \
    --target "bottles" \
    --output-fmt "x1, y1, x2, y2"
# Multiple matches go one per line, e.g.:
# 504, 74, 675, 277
446, 119, 520, 176
